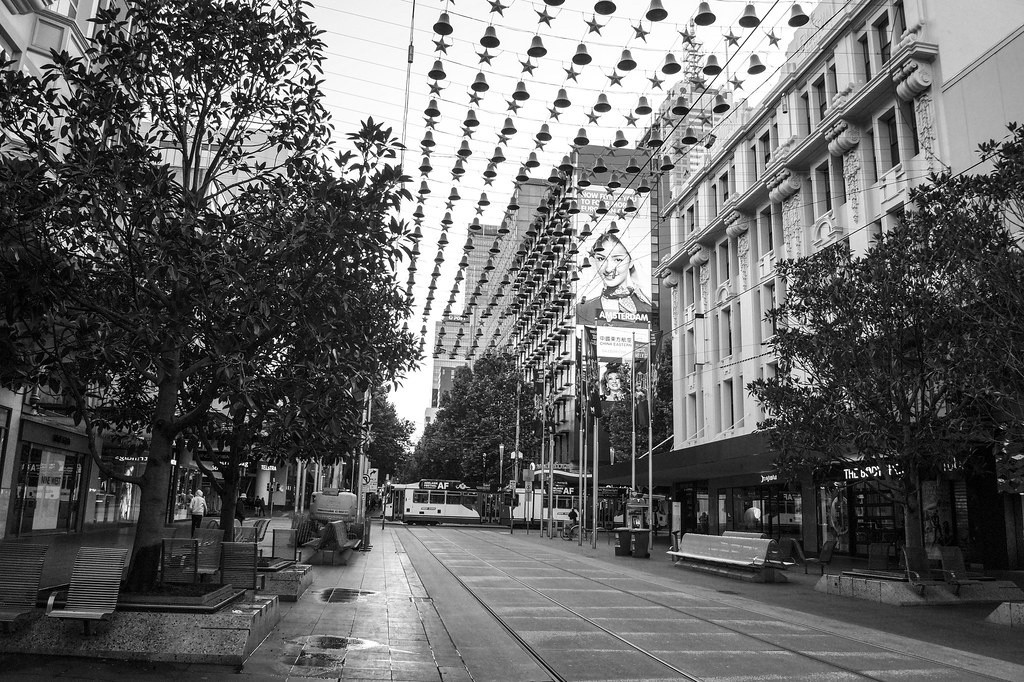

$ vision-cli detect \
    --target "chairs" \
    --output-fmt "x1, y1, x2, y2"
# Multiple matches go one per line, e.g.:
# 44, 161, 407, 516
813, 540, 839, 577
937, 546, 985, 597
234, 526, 263, 557
790, 537, 817, 574
243, 517, 272, 543
181, 528, 226, 582
158, 528, 179, 564
861, 542, 891, 571
161, 537, 207, 584
221, 541, 266, 591
45, 546, 128, 637
902, 545, 957, 598
272, 529, 302, 561
0, 542, 50, 631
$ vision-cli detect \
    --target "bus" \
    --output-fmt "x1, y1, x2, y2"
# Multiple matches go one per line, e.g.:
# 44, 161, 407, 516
381, 479, 545, 525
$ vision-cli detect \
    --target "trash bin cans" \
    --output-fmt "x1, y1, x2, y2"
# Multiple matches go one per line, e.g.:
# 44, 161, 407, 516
629, 529, 651, 558
614, 528, 633, 557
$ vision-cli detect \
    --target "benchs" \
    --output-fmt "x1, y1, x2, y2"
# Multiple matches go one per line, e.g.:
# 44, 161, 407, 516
664, 531, 797, 584
300, 519, 362, 565
197, 517, 241, 545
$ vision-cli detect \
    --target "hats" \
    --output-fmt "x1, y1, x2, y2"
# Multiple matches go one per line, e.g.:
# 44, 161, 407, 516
239, 493, 247, 498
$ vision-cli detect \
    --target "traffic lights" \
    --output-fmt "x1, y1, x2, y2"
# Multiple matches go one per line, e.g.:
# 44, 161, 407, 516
276, 482, 281, 492
269, 487, 274, 492
266, 482, 270, 492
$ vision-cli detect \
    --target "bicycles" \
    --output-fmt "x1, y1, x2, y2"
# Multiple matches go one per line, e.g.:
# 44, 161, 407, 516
560, 523, 581, 540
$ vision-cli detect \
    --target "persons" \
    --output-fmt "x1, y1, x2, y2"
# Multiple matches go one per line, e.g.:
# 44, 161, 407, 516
569, 508, 577, 526
233, 492, 247, 526
259, 496, 267, 517
254, 495, 261, 516
576, 223, 653, 331
189, 490, 207, 537
599, 367, 627, 402
